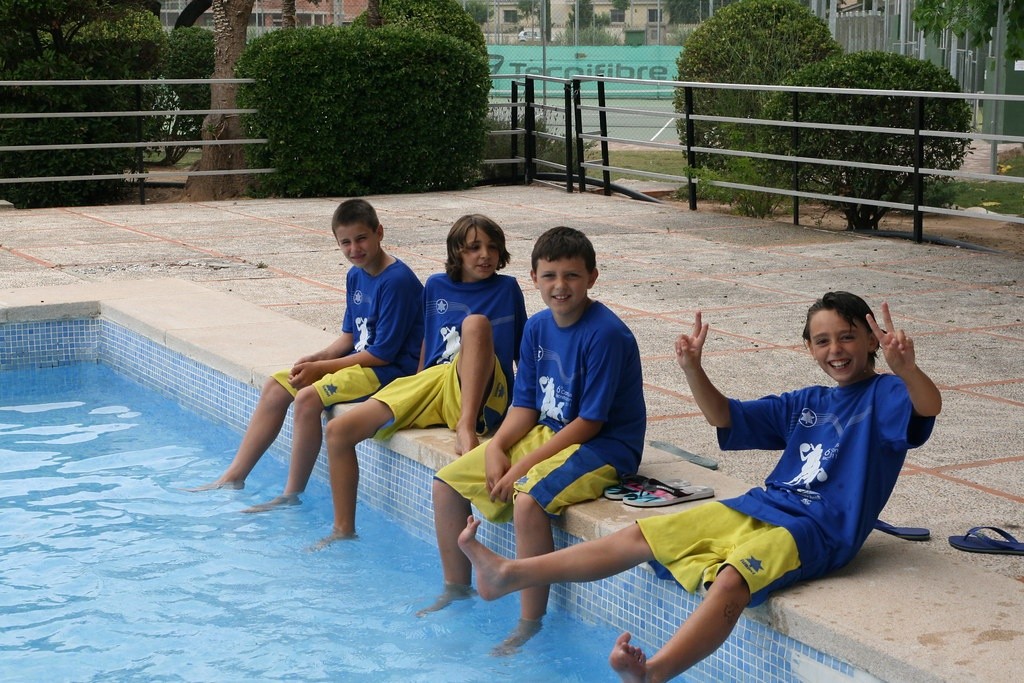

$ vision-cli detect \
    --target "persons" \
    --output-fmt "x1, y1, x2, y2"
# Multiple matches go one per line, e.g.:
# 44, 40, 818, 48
433, 225, 646, 621
326, 215, 528, 532
223, 199, 424, 490
458, 291, 942, 683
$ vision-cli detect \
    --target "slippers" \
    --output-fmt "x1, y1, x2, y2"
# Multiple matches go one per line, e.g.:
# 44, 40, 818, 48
873, 520, 929, 541
622, 478, 714, 507
604, 475, 691, 499
948, 527, 1024, 555
